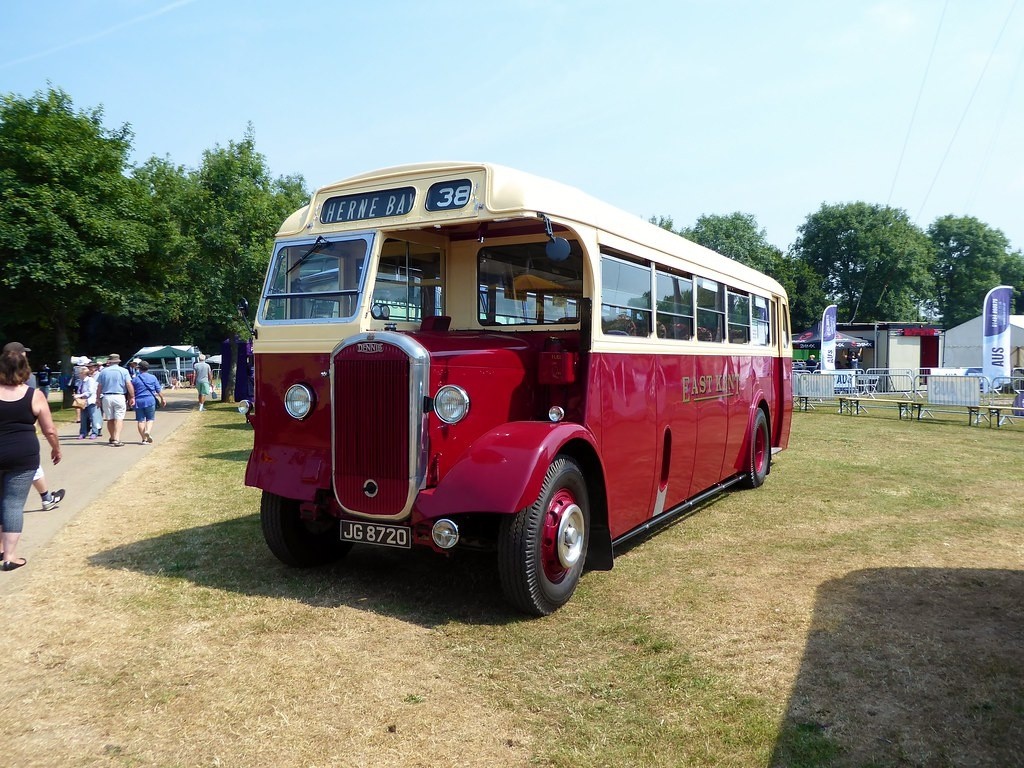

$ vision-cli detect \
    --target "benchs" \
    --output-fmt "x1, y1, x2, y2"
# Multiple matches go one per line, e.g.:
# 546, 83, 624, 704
792, 396, 1024, 428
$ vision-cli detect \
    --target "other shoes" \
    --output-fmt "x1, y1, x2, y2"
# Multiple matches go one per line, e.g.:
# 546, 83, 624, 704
142, 440, 146, 445
0, 552, 5, 562
113, 440, 125, 446
198, 406, 202, 412
91, 435, 96, 439
78, 435, 84, 440
203, 407, 207, 411
2, 558, 27, 570
109, 437, 113, 445
144, 431, 154, 443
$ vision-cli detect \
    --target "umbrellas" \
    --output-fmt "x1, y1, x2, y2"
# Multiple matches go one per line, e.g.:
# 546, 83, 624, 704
205, 355, 222, 383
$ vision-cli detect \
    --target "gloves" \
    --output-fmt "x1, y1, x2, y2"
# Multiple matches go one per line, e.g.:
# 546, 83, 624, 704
71, 394, 82, 400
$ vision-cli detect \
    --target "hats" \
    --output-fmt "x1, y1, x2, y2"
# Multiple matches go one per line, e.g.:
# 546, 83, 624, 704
136, 360, 149, 369
128, 358, 142, 367
3, 342, 31, 354
86, 360, 97, 368
107, 354, 122, 363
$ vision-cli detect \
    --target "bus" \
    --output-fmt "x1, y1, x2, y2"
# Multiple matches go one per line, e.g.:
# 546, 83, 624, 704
238, 161, 794, 616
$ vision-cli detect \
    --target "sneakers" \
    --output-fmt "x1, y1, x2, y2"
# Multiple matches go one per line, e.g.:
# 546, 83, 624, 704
41, 488, 65, 511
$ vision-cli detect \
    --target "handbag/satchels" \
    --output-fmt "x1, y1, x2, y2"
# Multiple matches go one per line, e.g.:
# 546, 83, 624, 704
211, 390, 218, 399
155, 398, 162, 408
72, 399, 88, 410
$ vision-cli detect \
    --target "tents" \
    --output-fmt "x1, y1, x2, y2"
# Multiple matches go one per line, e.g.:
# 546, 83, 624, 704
122, 346, 200, 388
792, 321, 875, 350
943, 315, 1023, 367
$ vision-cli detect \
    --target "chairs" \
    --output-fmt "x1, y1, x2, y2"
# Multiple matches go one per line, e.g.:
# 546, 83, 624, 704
556, 317, 746, 343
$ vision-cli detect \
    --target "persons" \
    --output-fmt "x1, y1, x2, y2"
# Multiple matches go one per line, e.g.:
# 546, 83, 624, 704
806, 354, 818, 373
842, 348, 862, 369
192, 354, 212, 411
37, 362, 51, 400
169, 373, 183, 389
0, 351, 62, 572
71, 354, 166, 447
3, 342, 66, 511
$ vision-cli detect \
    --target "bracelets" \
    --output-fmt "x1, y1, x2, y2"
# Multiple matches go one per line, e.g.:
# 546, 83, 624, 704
162, 398, 165, 400
